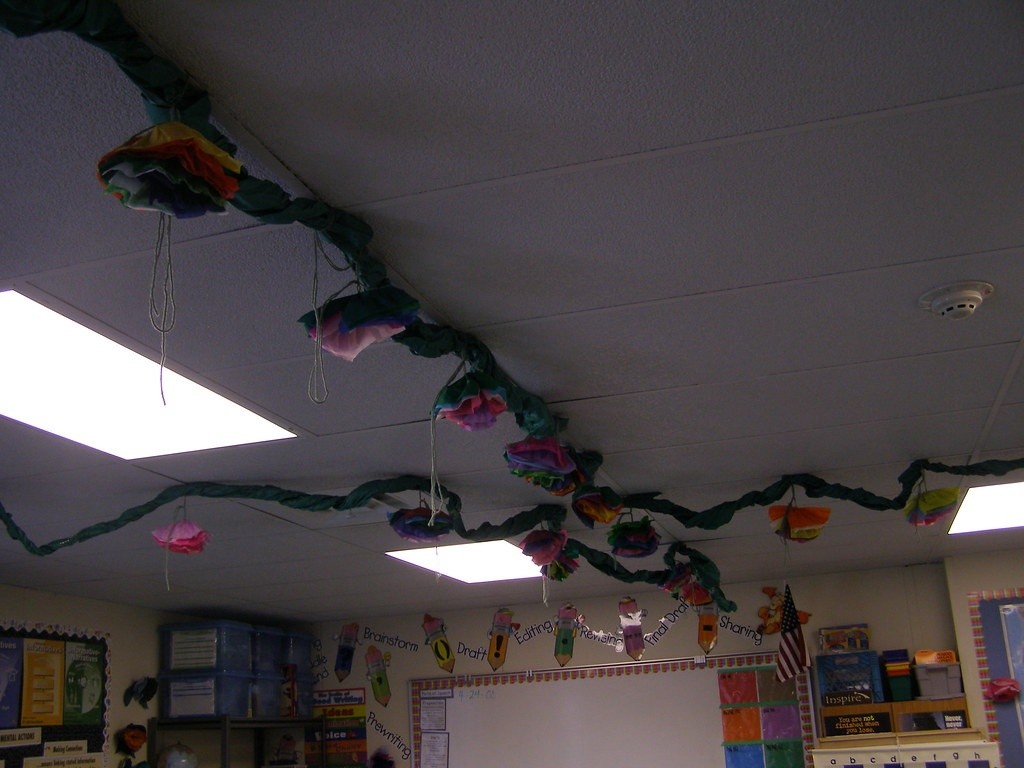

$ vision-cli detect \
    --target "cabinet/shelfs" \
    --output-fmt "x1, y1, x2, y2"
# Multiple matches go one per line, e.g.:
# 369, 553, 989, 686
811, 692, 1001, 768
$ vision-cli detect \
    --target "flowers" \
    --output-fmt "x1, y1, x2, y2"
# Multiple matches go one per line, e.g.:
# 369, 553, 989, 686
659, 563, 714, 607
115, 725, 147, 758
151, 519, 212, 555
296, 288, 420, 364
518, 529, 569, 566
605, 517, 666, 559
430, 371, 509, 432
93, 121, 240, 219
902, 486, 961, 525
388, 500, 454, 543
571, 486, 626, 528
503, 437, 583, 497
539, 553, 579, 583
767, 504, 832, 542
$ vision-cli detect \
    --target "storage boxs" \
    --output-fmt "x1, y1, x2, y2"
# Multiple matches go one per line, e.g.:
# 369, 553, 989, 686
814, 650, 883, 703
157, 617, 314, 721
914, 663, 962, 697
887, 674, 914, 701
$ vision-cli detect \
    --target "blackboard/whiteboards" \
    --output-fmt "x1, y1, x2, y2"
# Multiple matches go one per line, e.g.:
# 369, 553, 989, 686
407, 648, 818, 768
